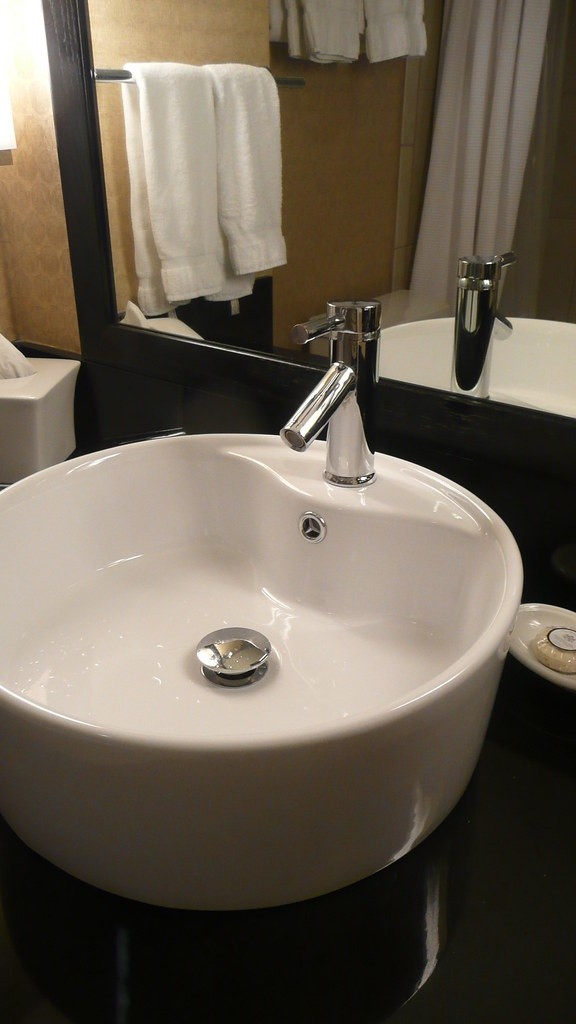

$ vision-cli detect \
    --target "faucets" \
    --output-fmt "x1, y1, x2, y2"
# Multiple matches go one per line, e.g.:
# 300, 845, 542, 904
277, 296, 382, 490
448, 250, 523, 400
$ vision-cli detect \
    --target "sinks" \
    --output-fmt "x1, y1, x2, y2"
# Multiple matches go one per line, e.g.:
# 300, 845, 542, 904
374, 314, 576, 419
0, 434, 525, 911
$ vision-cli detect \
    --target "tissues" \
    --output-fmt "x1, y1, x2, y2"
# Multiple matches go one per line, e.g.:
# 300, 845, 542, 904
117, 298, 206, 343
0, 332, 82, 485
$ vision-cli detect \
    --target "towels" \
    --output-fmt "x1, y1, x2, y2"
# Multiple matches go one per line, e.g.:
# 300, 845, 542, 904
121, 60, 290, 318
266, 0, 430, 66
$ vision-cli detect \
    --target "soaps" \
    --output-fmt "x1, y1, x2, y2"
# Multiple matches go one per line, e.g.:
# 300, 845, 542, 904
530, 623, 576, 675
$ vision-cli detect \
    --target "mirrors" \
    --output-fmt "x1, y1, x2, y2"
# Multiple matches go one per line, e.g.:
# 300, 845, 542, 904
42, 0, 576, 469
